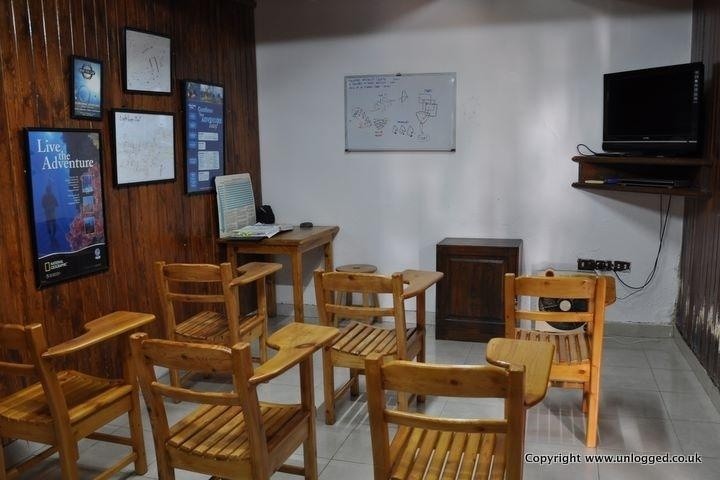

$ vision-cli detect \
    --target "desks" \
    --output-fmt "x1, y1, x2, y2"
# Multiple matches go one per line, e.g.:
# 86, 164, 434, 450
215, 223, 340, 322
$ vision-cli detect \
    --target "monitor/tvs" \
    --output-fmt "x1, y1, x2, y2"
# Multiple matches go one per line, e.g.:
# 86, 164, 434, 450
601, 61, 706, 153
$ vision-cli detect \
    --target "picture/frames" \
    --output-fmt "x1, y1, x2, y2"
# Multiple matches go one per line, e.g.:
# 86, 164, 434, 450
181, 79, 225, 194
110, 107, 177, 188
122, 27, 173, 96
21, 126, 110, 290
69, 54, 103, 122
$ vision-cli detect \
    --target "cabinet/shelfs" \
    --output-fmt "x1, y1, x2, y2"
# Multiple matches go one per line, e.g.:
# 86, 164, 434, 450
436, 237, 523, 343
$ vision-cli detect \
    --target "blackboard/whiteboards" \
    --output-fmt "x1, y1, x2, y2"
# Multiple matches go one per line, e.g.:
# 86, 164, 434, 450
343, 72, 457, 152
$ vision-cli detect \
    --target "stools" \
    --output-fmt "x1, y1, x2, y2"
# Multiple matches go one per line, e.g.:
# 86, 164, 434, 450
333, 264, 382, 327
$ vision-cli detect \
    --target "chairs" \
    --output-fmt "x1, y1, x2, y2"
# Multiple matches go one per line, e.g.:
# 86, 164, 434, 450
129, 322, 341, 480
153, 262, 283, 403
0, 311, 155, 480
365, 337, 554, 480
504, 272, 607, 448
314, 269, 444, 424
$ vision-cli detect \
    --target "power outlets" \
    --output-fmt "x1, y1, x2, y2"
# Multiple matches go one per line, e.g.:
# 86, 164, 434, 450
596, 260, 611, 270
577, 259, 595, 270
615, 261, 630, 272
